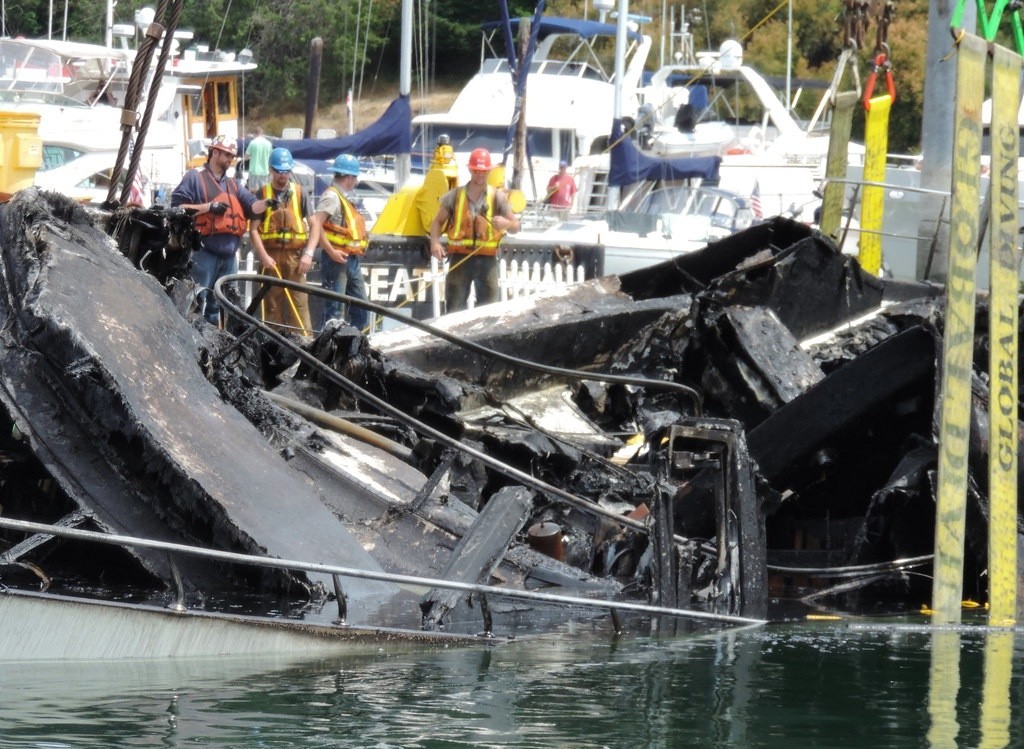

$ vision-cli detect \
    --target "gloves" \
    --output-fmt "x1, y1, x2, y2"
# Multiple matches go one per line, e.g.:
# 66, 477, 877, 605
210, 202, 233, 214
265, 199, 281, 210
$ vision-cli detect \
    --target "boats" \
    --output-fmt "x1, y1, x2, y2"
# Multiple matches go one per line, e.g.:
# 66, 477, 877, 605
1, 1, 1024, 676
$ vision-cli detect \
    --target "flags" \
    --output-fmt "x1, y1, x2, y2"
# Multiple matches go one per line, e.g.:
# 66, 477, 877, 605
750, 181, 765, 219
128, 131, 148, 206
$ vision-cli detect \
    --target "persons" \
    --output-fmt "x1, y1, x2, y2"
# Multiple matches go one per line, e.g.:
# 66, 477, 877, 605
427, 146, 524, 314
244, 125, 273, 193
249, 147, 322, 341
430, 131, 460, 193
545, 159, 578, 208
171, 133, 282, 315
317, 153, 371, 339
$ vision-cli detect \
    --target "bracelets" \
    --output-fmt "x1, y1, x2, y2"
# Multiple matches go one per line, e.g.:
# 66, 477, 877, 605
303, 248, 314, 259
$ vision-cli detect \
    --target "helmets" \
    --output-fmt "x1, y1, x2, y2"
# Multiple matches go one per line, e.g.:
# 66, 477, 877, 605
269, 148, 297, 173
206, 134, 237, 156
466, 149, 492, 172
327, 154, 359, 177
